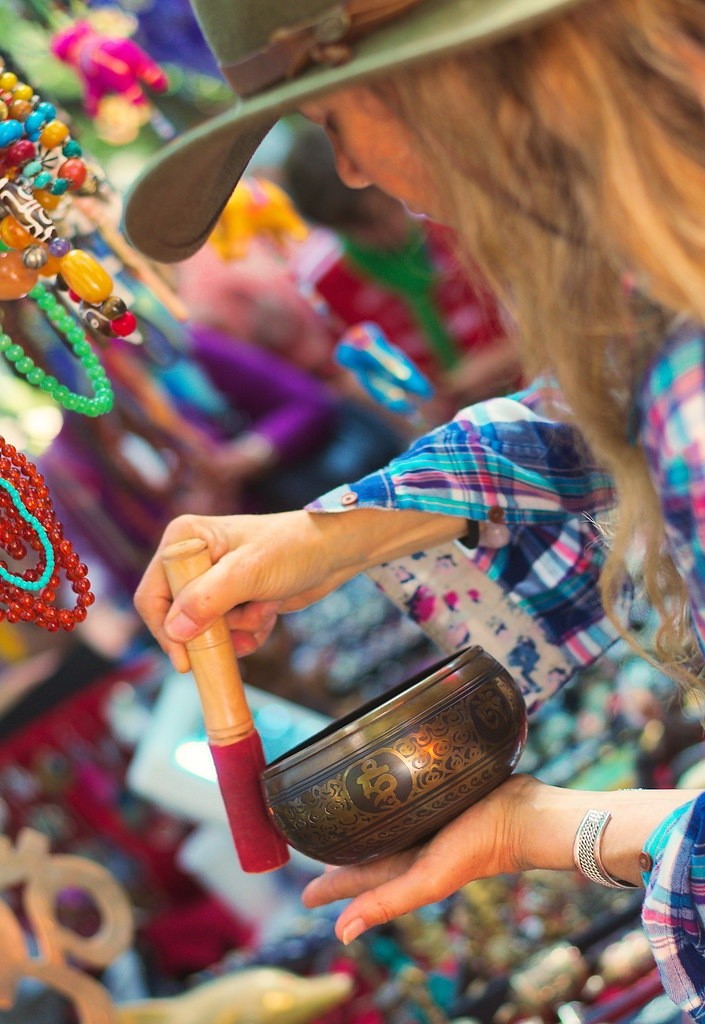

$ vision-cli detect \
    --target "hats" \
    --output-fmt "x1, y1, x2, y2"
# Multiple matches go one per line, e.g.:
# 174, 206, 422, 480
120, 0, 574, 264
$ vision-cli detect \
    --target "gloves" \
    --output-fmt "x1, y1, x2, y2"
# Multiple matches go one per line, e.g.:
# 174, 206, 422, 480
209, 176, 308, 262
334, 326, 432, 416
49, 23, 167, 120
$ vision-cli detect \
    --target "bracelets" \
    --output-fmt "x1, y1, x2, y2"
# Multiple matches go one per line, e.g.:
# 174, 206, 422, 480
572, 789, 644, 889
460, 521, 483, 549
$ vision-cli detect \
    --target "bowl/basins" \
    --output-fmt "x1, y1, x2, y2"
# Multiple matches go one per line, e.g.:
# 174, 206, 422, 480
260, 644, 529, 866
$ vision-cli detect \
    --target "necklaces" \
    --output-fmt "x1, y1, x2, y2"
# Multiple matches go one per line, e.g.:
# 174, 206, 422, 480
0, 47, 143, 633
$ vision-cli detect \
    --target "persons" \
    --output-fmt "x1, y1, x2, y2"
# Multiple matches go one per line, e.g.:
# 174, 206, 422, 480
126, 0, 705, 1024
